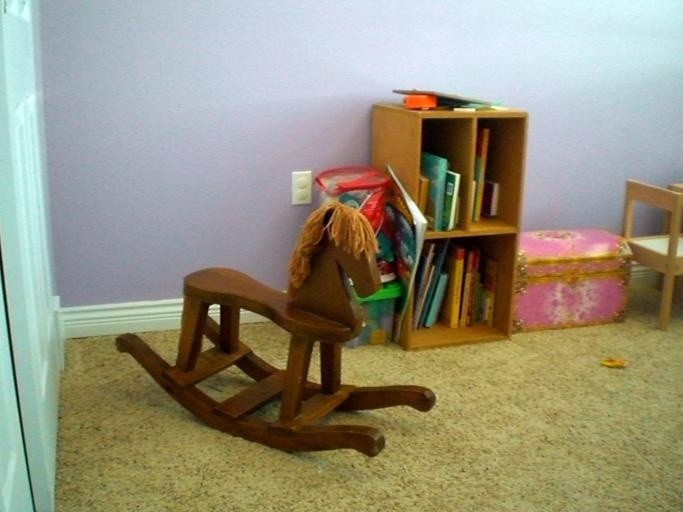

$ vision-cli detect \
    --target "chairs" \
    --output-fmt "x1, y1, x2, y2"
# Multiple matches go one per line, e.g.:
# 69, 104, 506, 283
622, 180, 683, 331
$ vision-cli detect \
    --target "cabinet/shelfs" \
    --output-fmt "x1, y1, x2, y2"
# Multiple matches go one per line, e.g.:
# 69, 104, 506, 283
369, 102, 529, 352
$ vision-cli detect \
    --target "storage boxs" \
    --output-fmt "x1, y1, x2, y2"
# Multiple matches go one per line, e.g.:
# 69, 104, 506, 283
345, 277, 402, 347
315, 165, 393, 231
510, 229, 633, 334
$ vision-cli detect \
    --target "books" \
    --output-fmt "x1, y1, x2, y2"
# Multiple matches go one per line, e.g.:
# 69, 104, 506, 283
414, 239, 497, 328
419, 153, 461, 231
472, 127, 498, 222
